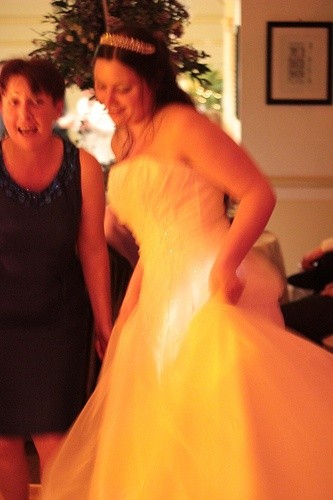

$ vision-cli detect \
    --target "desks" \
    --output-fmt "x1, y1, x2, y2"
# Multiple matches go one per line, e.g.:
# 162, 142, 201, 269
249, 230, 289, 307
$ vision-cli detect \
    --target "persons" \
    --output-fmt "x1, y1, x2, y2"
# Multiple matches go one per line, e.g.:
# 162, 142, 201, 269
0, 59, 114, 500
42, 19, 332, 500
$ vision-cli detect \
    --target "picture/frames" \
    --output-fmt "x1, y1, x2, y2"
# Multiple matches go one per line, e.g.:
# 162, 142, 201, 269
265, 21, 332, 107
